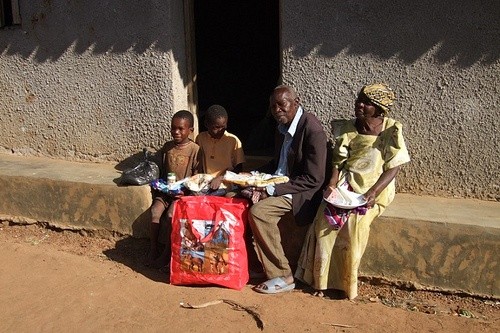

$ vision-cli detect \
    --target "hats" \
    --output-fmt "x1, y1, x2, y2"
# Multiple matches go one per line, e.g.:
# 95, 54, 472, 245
361, 83, 395, 114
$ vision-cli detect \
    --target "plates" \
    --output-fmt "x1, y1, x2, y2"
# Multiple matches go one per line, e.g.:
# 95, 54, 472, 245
323, 190, 368, 210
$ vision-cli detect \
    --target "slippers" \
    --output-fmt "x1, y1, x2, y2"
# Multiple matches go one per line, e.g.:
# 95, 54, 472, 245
254, 275, 296, 294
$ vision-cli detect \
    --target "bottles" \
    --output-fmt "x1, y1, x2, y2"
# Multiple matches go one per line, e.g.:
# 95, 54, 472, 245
167, 173, 177, 185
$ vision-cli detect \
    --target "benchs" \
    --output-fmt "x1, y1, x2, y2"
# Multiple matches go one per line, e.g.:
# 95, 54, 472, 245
0, 156, 500, 301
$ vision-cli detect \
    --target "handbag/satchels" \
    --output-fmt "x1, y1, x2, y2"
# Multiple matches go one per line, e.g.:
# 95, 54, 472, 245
168, 195, 251, 289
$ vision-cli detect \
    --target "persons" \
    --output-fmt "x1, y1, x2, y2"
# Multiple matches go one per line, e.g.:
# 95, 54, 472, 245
195, 105, 246, 197
227, 85, 326, 295
143, 110, 199, 272
294, 83, 410, 301
